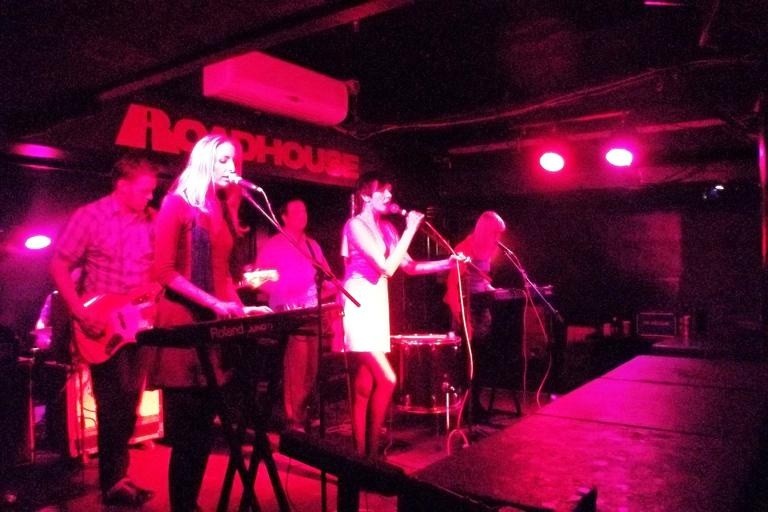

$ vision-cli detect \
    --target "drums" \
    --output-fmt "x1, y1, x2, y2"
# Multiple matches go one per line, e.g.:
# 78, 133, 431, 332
388, 333, 466, 416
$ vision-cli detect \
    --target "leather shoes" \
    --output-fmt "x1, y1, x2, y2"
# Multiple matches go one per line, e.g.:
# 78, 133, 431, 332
101, 480, 154, 507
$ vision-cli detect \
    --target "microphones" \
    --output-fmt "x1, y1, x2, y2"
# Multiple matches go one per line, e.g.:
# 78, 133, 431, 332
495, 241, 513, 255
388, 204, 406, 218
227, 172, 260, 192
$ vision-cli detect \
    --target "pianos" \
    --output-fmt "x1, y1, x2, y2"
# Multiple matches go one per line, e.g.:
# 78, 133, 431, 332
135, 302, 344, 346
461, 283, 553, 304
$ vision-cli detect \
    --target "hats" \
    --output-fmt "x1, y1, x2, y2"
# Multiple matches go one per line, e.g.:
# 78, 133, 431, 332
354, 171, 400, 195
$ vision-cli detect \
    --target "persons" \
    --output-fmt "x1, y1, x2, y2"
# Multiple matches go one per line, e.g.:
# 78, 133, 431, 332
437, 211, 505, 415
339, 171, 472, 464
254, 194, 331, 443
143, 133, 278, 512
45, 156, 155, 505
